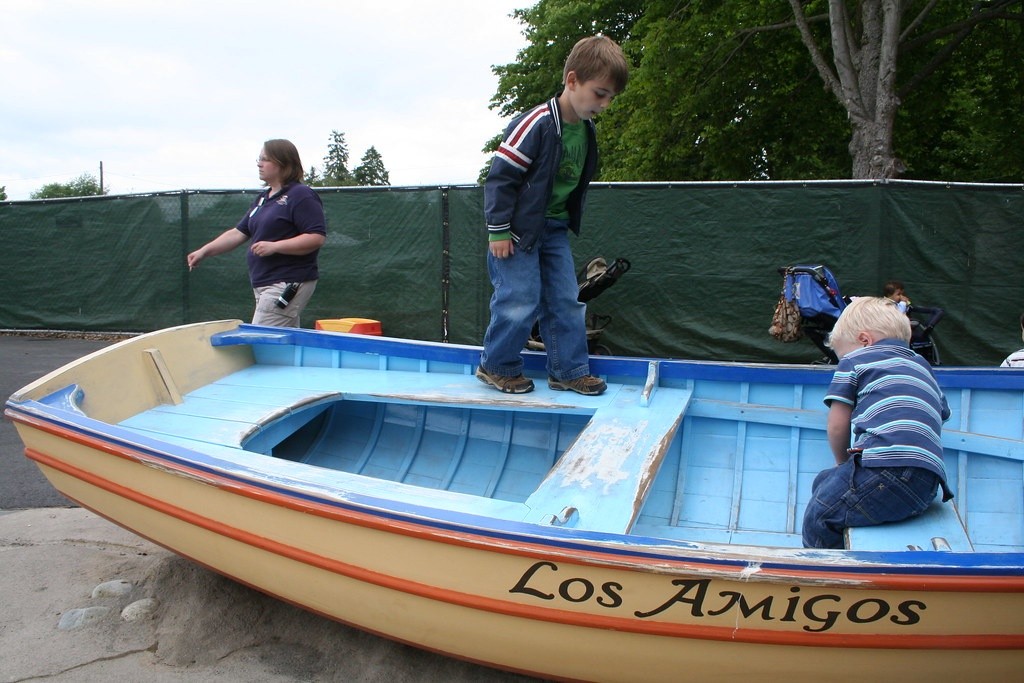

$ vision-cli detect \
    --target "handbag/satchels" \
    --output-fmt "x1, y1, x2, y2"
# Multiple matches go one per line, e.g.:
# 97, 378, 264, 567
768, 267, 802, 342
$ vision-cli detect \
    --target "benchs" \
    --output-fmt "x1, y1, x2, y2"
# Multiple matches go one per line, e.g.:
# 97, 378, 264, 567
840, 486, 976, 551
522, 383, 693, 535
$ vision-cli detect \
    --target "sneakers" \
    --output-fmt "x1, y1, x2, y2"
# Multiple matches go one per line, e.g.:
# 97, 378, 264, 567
548, 372, 607, 394
475, 362, 534, 393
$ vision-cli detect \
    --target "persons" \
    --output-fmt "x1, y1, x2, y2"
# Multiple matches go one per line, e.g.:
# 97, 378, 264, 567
187, 139, 327, 329
802, 296, 954, 551
1000, 313, 1024, 368
475, 35, 628, 395
884, 280, 936, 344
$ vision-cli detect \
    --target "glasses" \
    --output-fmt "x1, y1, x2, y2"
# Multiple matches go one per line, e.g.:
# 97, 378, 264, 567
256, 157, 270, 163
249, 195, 267, 218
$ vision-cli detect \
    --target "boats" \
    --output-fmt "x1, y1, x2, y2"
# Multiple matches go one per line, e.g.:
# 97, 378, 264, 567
2, 316, 1023, 683
